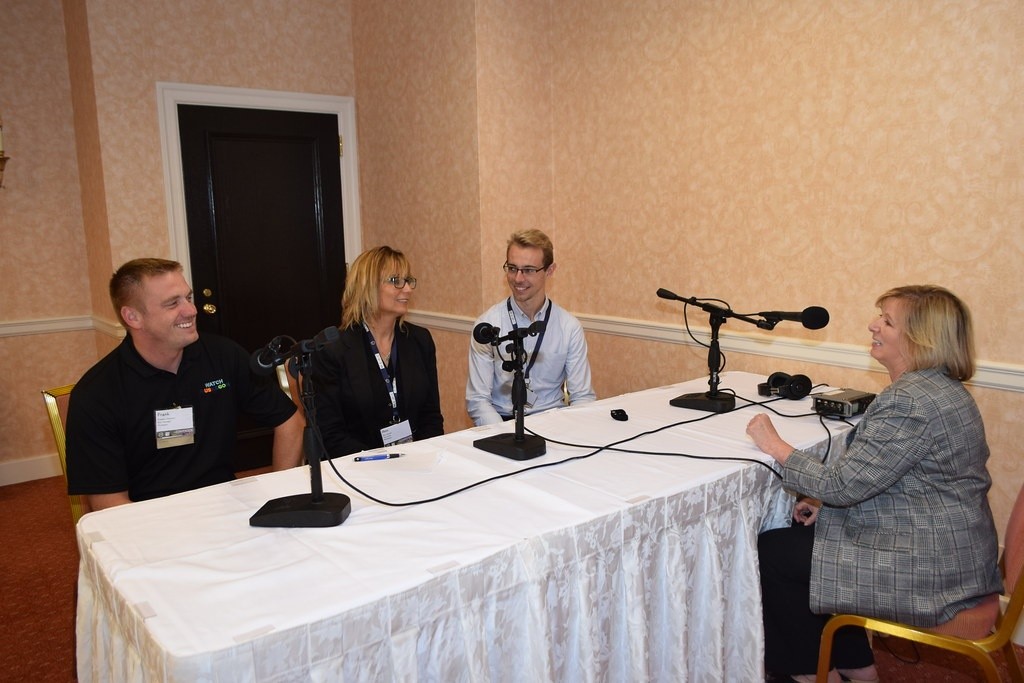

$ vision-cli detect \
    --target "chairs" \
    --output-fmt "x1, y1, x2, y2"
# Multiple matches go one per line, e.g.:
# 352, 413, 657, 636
277, 357, 308, 467
813, 483, 1024, 683
41, 382, 93, 526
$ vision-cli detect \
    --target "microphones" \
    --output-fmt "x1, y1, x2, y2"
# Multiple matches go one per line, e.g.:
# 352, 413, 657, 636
248, 336, 283, 376
758, 306, 830, 329
473, 322, 500, 344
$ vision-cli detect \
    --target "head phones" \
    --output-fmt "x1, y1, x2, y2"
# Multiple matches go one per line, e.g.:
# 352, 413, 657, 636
757, 372, 812, 400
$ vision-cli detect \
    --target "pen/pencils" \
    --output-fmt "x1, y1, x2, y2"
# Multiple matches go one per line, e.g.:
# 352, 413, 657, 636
354, 453, 405, 461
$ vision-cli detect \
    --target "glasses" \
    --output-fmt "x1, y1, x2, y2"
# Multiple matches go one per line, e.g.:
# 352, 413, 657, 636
503, 261, 545, 275
380, 277, 417, 289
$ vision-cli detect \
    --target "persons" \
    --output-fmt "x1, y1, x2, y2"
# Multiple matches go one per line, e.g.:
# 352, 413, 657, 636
745, 284, 1006, 683
465, 229, 597, 428
300, 246, 445, 461
66, 258, 304, 511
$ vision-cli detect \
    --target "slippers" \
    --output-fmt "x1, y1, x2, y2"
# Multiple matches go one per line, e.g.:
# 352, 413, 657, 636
766, 674, 801, 683
838, 672, 880, 683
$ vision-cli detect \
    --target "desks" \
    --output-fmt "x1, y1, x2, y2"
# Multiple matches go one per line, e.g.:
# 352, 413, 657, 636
75, 371, 862, 683
